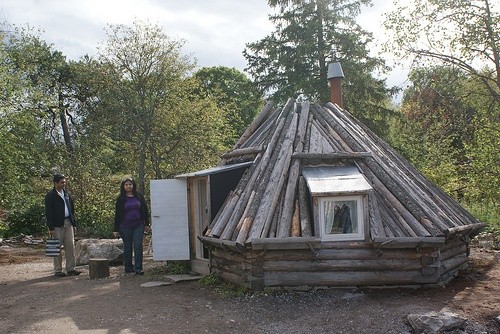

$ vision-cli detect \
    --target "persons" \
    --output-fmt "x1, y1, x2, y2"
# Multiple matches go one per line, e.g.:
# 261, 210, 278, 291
113, 176, 150, 274
45, 173, 81, 276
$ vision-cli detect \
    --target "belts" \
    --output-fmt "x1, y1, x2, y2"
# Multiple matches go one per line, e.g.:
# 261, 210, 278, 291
64, 216, 69, 218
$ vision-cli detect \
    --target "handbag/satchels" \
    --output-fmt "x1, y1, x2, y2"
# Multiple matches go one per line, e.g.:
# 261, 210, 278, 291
45, 234, 61, 257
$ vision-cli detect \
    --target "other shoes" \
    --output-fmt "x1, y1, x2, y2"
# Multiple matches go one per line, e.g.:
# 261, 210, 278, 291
56, 272, 65, 276
136, 270, 144, 274
68, 270, 80, 275
